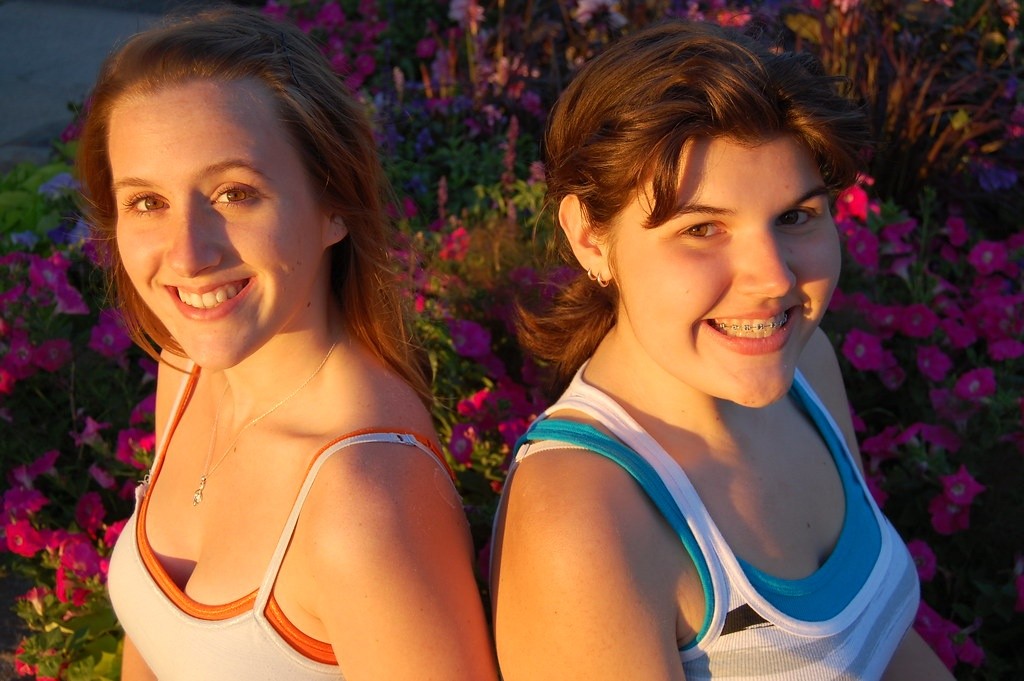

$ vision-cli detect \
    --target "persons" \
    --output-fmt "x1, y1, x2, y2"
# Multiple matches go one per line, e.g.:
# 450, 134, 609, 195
80, 11, 494, 681
494, 27, 959, 681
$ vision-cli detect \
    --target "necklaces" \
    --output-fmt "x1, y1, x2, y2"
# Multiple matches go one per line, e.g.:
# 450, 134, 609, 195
194, 323, 348, 504
